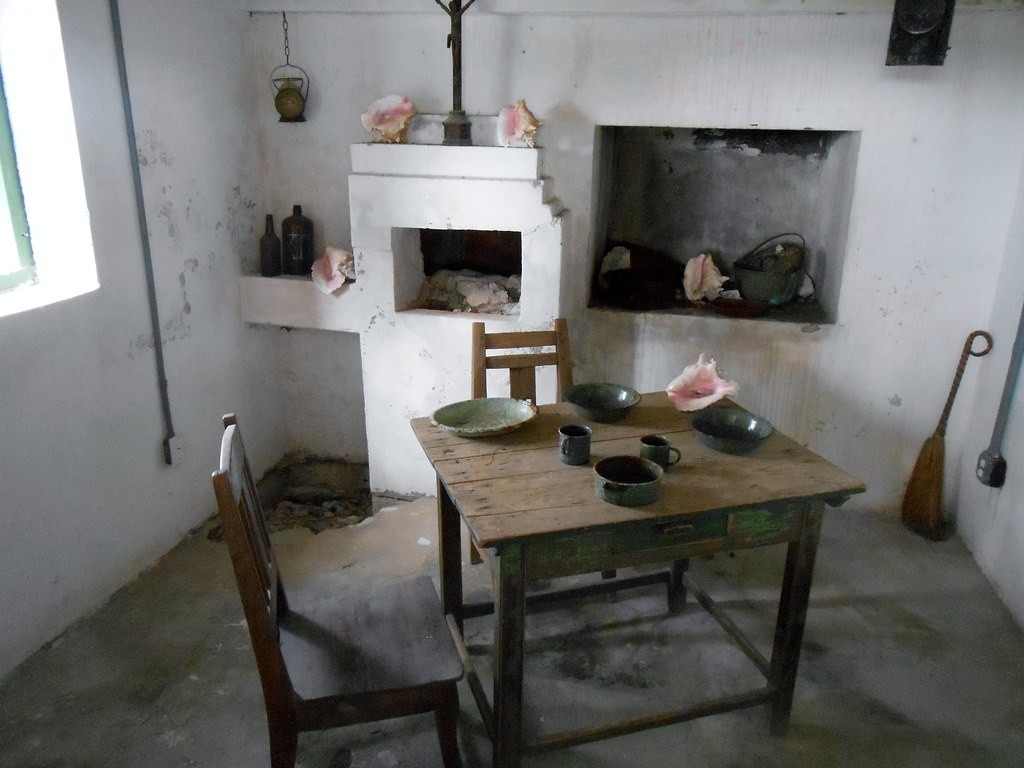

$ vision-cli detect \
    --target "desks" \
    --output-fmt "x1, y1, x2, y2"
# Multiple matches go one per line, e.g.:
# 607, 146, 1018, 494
410, 392, 867, 768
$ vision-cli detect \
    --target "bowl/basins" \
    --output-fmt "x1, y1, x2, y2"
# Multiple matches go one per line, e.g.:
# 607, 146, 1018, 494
562, 383, 642, 422
591, 454, 663, 508
689, 407, 774, 452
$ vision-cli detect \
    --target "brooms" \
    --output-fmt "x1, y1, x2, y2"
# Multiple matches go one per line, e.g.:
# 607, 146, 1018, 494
899, 331, 993, 542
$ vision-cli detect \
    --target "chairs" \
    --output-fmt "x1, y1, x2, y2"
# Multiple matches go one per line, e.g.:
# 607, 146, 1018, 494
471, 319, 617, 602
213, 413, 466, 768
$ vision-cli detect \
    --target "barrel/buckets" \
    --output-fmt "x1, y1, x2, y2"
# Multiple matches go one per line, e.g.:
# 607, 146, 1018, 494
733, 261, 801, 306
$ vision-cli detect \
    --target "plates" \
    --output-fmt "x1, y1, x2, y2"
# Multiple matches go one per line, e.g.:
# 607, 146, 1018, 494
430, 398, 539, 435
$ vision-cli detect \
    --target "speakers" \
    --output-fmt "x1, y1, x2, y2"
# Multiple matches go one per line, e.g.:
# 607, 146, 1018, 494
885, 0, 956, 66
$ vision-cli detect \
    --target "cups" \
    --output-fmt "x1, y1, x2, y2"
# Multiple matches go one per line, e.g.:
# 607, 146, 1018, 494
640, 434, 682, 467
558, 425, 592, 466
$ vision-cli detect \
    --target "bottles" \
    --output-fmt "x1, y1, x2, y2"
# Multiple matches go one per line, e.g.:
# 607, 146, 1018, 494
259, 213, 281, 277
283, 205, 315, 274
